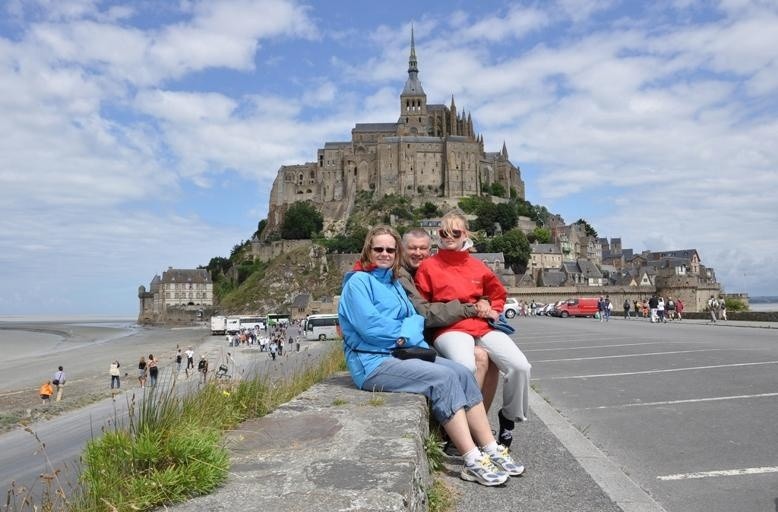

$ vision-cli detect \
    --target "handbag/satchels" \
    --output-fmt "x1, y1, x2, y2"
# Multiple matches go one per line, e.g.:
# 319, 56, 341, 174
608, 303, 613, 310
390, 345, 437, 363
53, 380, 59, 385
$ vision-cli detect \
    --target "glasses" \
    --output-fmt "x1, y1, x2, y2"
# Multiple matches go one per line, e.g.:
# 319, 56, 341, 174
438, 228, 468, 240
369, 244, 398, 255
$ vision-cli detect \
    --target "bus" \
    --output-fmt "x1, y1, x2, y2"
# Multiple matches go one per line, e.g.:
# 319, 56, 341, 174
210, 313, 291, 337
304, 313, 342, 342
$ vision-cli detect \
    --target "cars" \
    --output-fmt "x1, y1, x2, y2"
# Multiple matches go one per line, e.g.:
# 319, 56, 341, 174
504, 296, 600, 319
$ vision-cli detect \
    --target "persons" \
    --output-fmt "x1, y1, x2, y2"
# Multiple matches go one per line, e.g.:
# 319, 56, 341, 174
39, 377, 54, 404
518, 299, 524, 317
53, 366, 67, 401
717, 294, 728, 321
109, 359, 121, 390
414, 210, 533, 459
705, 294, 718, 324
175, 348, 182, 373
336, 224, 528, 488
147, 354, 158, 387
197, 355, 207, 383
524, 301, 529, 316
224, 351, 236, 378
395, 226, 501, 415
224, 316, 305, 360
530, 299, 537, 316
184, 346, 194, 368
596, 294, 684, 324
138, 355, 147, 389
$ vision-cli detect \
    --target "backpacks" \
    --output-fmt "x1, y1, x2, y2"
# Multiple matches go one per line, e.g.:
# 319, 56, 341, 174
720, 300, 726, 310
667, 301, 674, 306
710, 299, 717, 309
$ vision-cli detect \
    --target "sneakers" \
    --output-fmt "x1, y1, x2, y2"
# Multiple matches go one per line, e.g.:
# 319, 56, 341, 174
482, 445, 526, 477
498, 408, 514, 447
460, 455, 509, 488
